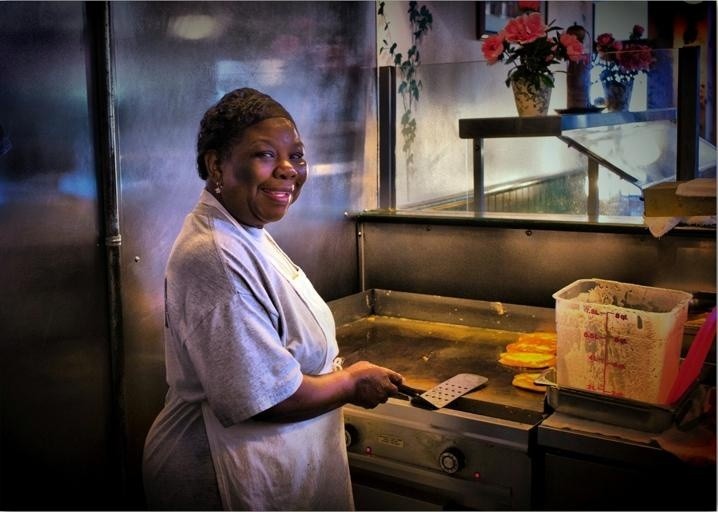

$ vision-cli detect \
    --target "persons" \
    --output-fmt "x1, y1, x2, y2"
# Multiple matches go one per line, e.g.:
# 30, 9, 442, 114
139, 86, 403, 510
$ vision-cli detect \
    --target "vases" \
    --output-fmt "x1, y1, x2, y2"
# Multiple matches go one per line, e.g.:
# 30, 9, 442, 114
510, 54, 636, 117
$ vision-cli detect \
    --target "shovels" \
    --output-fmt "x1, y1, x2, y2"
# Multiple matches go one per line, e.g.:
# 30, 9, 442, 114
391, 372, 488, 410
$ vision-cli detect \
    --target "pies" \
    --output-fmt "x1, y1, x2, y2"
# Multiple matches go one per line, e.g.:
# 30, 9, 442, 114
499, 333, 556, 392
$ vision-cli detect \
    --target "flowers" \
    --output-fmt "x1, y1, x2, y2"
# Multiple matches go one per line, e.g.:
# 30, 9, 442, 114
480, 10, 660, 91
